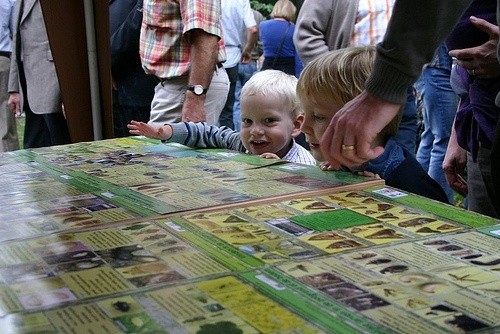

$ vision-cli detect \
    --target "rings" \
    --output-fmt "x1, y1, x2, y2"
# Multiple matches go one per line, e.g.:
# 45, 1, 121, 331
342, 144, 355, 150
472, 69, 475, 76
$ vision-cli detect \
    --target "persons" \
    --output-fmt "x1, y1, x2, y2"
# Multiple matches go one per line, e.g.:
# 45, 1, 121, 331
0, 0, 500, 219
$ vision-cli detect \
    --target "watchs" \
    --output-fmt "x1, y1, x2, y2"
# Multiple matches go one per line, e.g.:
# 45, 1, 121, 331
186, 84, 207, 96
